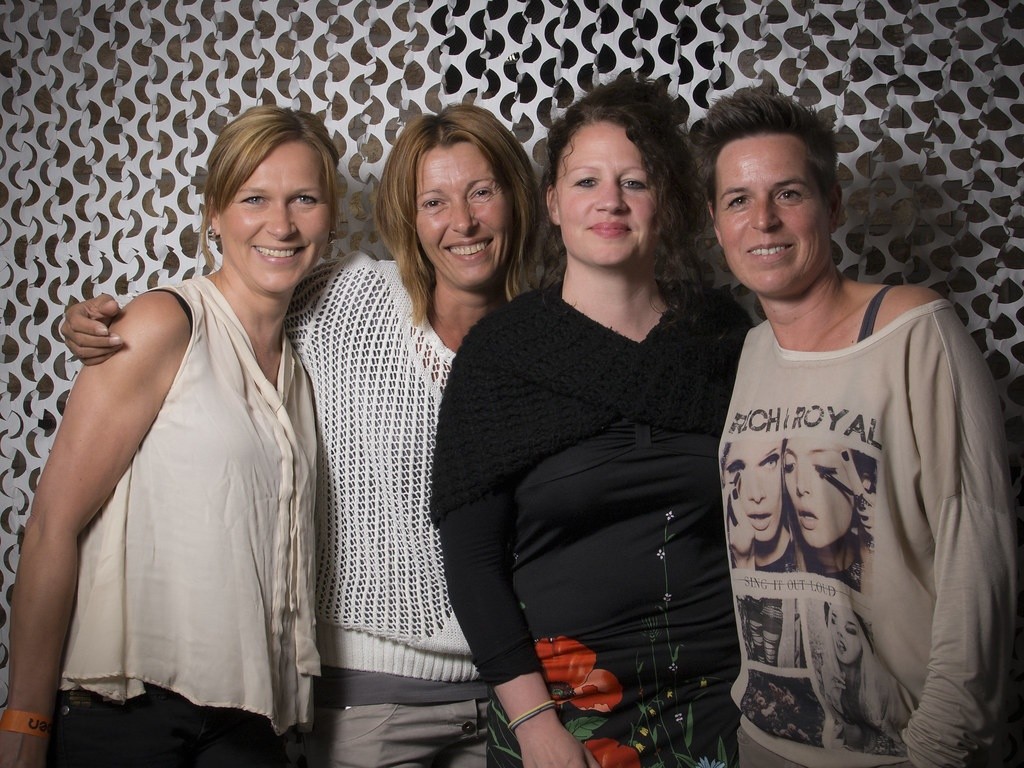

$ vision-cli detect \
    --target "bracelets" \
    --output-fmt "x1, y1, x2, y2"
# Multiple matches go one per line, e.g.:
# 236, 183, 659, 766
0, 709, 52, 738
508, 699, 557, 735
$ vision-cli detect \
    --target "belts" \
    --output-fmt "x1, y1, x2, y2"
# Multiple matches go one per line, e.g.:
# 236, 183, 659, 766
56, 692, 186, 707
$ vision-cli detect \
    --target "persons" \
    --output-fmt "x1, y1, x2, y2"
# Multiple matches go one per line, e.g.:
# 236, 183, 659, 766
721, 436, 874, 595
1, 106, 341, 768
699, 94, 1019, 768
430, 76, 756, 768
746, 598, 905, 754
58, 105, 537, 768
